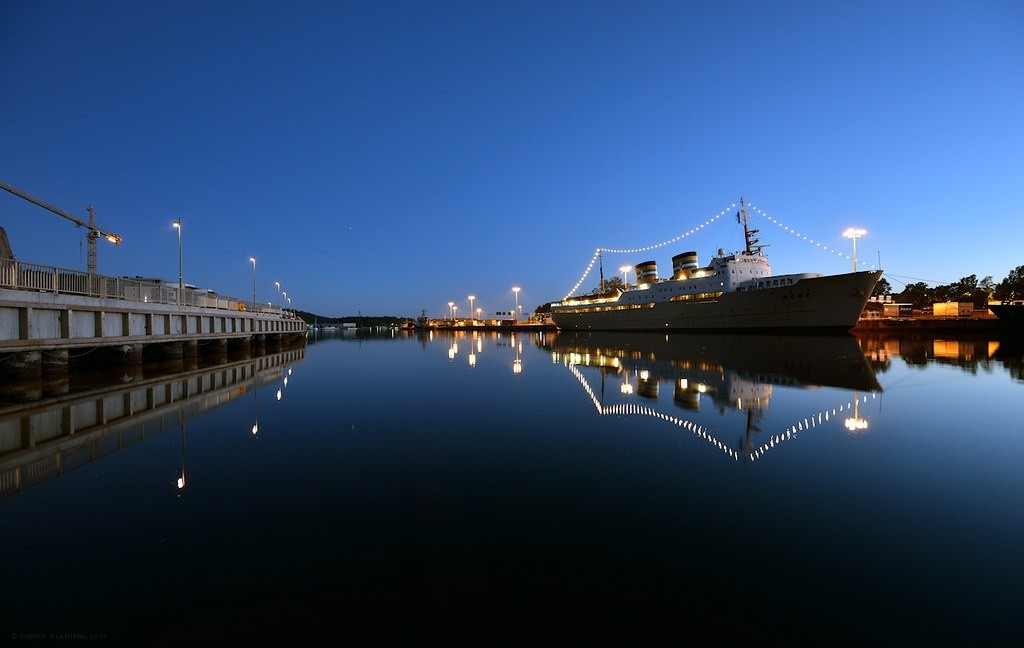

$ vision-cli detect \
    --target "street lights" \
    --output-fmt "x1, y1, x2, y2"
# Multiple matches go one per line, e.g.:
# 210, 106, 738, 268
282, 292, 287, 319
452, 306, 458, 320
250, 257, 258, 303
172, 220, 182, 305
275, 282, 282, 317
476, 308, 482, 320
448, 302, 454, 320
468, 295, 476, 320
512, 288, 520, 319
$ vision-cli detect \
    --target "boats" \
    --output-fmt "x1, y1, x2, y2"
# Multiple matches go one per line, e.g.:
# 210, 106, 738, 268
540, 331, 887, 462
985, 302, 1024, 329
553, 197, 884, 332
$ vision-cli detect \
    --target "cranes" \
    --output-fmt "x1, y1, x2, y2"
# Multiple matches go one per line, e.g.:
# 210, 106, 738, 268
0, 182, 123, 276
